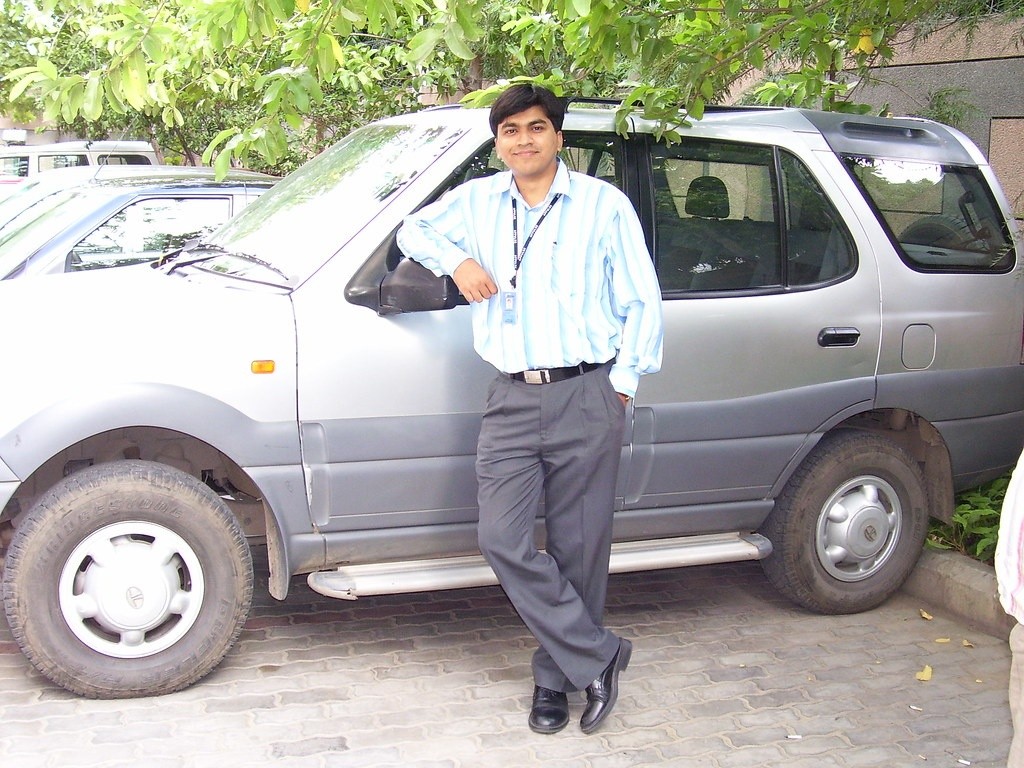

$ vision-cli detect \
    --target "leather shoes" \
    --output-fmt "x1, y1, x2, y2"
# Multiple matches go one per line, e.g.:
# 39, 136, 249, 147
580, 637, 633, 734
528, 683, 571, 735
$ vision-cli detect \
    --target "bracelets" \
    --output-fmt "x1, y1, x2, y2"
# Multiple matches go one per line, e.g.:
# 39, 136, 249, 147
621, 394, 630, 401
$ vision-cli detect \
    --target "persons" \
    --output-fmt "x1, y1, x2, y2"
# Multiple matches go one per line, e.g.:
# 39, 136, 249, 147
395, 82, 662, 734
506, 299, 513, 309
994, 446, 1024, 768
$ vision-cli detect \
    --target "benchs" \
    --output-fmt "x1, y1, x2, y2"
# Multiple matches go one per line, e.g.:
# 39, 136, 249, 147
662, 176, 841, 292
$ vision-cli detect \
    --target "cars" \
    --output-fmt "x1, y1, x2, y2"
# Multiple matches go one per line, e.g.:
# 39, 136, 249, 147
0, 138, 285, 282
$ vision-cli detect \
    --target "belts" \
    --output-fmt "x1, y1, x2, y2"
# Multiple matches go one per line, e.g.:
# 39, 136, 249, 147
508, 360, 601, 385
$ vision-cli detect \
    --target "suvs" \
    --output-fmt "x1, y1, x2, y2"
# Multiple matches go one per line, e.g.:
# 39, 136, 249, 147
0, 94, 1024, 700
